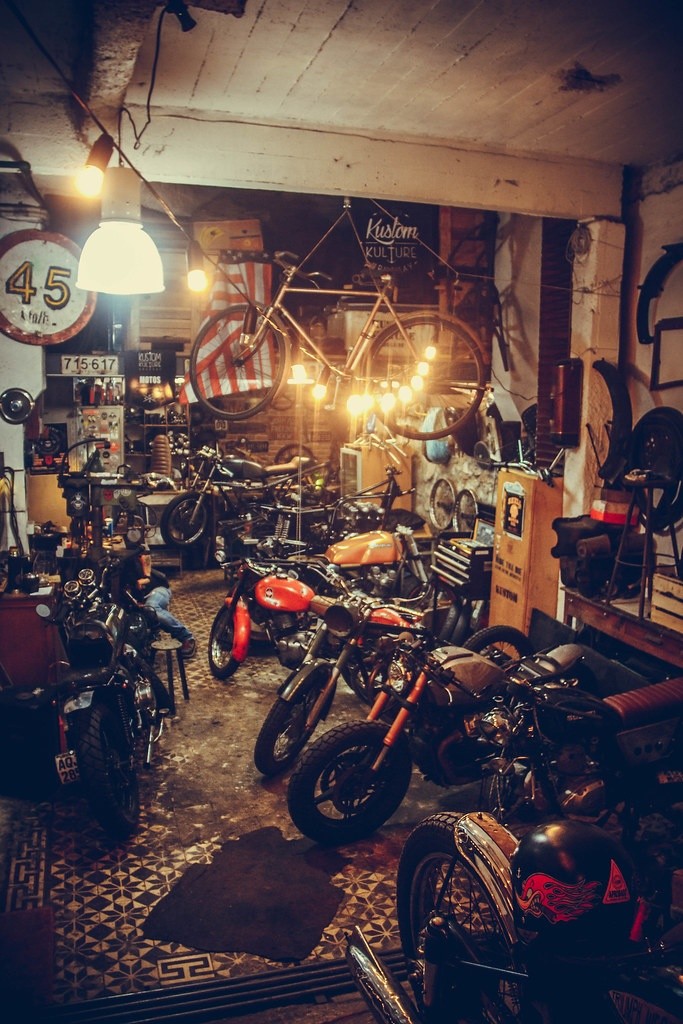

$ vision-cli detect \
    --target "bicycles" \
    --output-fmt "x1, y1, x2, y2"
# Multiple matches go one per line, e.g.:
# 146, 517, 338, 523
189, 251, 493, 439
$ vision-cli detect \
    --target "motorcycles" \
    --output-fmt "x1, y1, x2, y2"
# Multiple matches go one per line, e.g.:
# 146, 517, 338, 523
33, 547, 171, 837
209, 507, 683, 1024
159, 442, 324, 546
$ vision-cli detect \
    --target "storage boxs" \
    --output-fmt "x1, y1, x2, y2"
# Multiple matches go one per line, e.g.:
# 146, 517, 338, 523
192, 218, 263, 250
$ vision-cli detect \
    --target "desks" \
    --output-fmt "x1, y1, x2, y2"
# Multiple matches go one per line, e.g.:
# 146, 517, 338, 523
560, 587, 683, 668
137, 490, 187, 578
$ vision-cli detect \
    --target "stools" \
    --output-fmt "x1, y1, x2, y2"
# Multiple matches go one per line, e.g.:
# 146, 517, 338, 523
151, 639, 190, 702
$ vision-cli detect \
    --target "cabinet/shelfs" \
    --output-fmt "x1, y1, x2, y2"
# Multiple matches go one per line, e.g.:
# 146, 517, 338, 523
0, 584, 61, 687
125, 388, 191, 479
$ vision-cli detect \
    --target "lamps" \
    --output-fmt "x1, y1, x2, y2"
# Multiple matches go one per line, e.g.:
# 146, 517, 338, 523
185, 240, 210, 293
73, 106, 166, 295
77, 132, 115, 199
